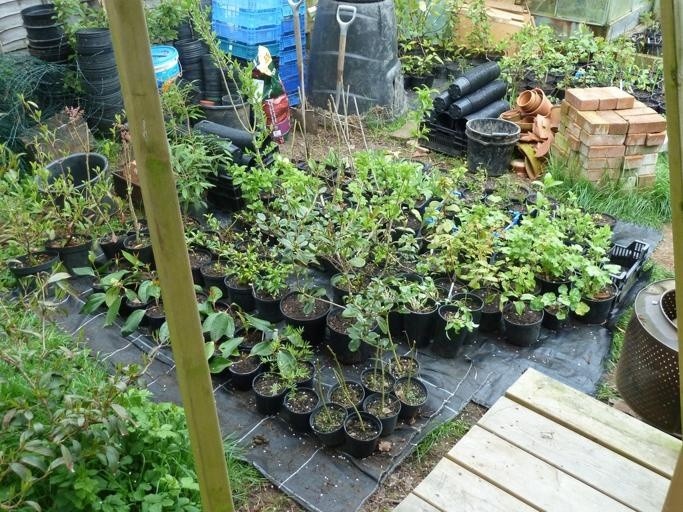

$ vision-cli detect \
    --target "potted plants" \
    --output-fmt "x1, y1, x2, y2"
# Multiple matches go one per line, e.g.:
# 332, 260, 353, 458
498, 10, 665, 116
393, 1, 502, 91
90, 141, 619, 460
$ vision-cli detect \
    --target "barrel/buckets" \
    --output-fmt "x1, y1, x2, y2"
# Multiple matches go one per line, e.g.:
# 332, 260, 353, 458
33, 152, 115, 222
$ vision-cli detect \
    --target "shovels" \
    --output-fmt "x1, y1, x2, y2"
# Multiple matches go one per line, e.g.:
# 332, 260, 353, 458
288, 0, 318, 135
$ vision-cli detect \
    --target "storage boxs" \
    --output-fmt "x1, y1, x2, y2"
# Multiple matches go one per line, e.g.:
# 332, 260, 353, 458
201, 141, 280, 211
417, 121, 466, 160
211, 0, 308, 106
572, 239, 649, 308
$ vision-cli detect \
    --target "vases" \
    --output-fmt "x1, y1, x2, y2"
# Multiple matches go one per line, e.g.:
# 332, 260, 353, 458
497, 87, 560, 175
424, 61, 510, 133
174, 37, 251, 131
168, 119, 271, 175
0, 161, 59, 302
74, 27, 127, 144
35, 151, 117, 235
20, 3, 79, 113
